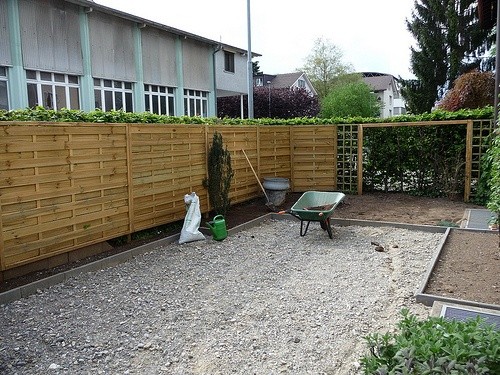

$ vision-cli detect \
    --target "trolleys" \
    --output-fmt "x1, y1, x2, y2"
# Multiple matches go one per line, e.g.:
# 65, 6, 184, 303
279, 190, 346, 240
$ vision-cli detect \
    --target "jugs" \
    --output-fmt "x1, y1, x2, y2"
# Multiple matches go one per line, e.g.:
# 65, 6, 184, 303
204, 214, 228, 240
